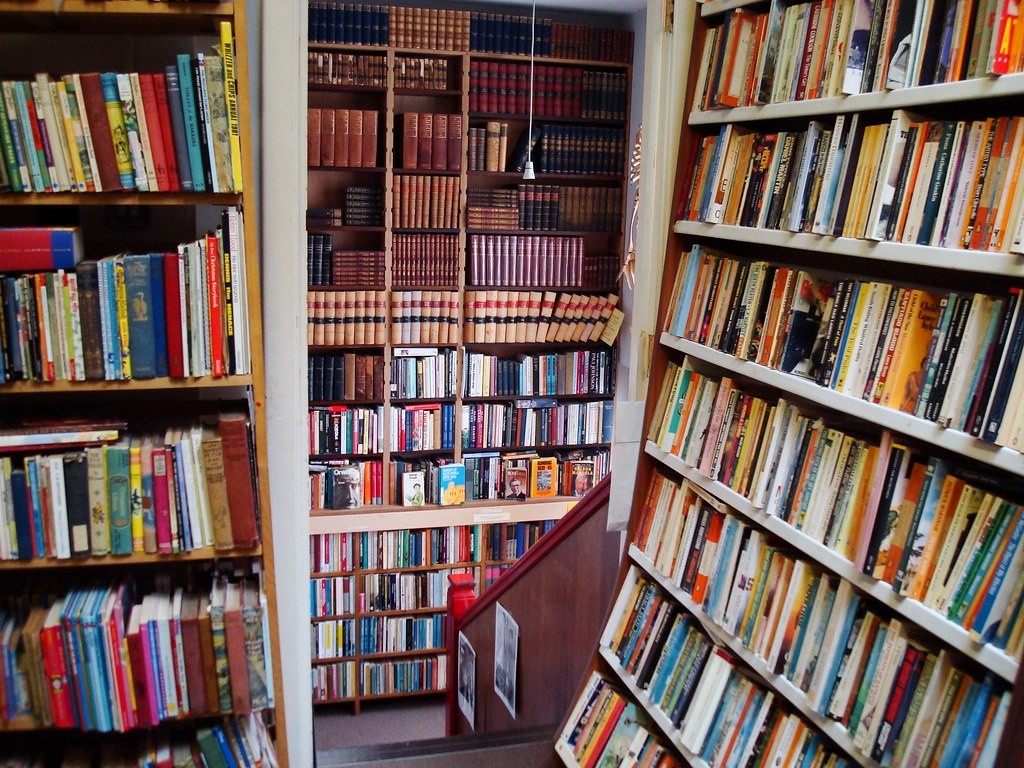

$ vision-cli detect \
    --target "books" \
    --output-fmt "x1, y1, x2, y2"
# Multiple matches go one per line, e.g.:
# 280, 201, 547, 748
553, 0, 1024, 768
308, 2, 626, 703
0, 21, 280, 768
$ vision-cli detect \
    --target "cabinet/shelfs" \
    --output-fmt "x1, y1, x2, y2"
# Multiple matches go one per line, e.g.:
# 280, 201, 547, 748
310, 521, 545, 716
553, 0, 1024, 768
0, 0, 290, 768
306, 40, 634, 517
